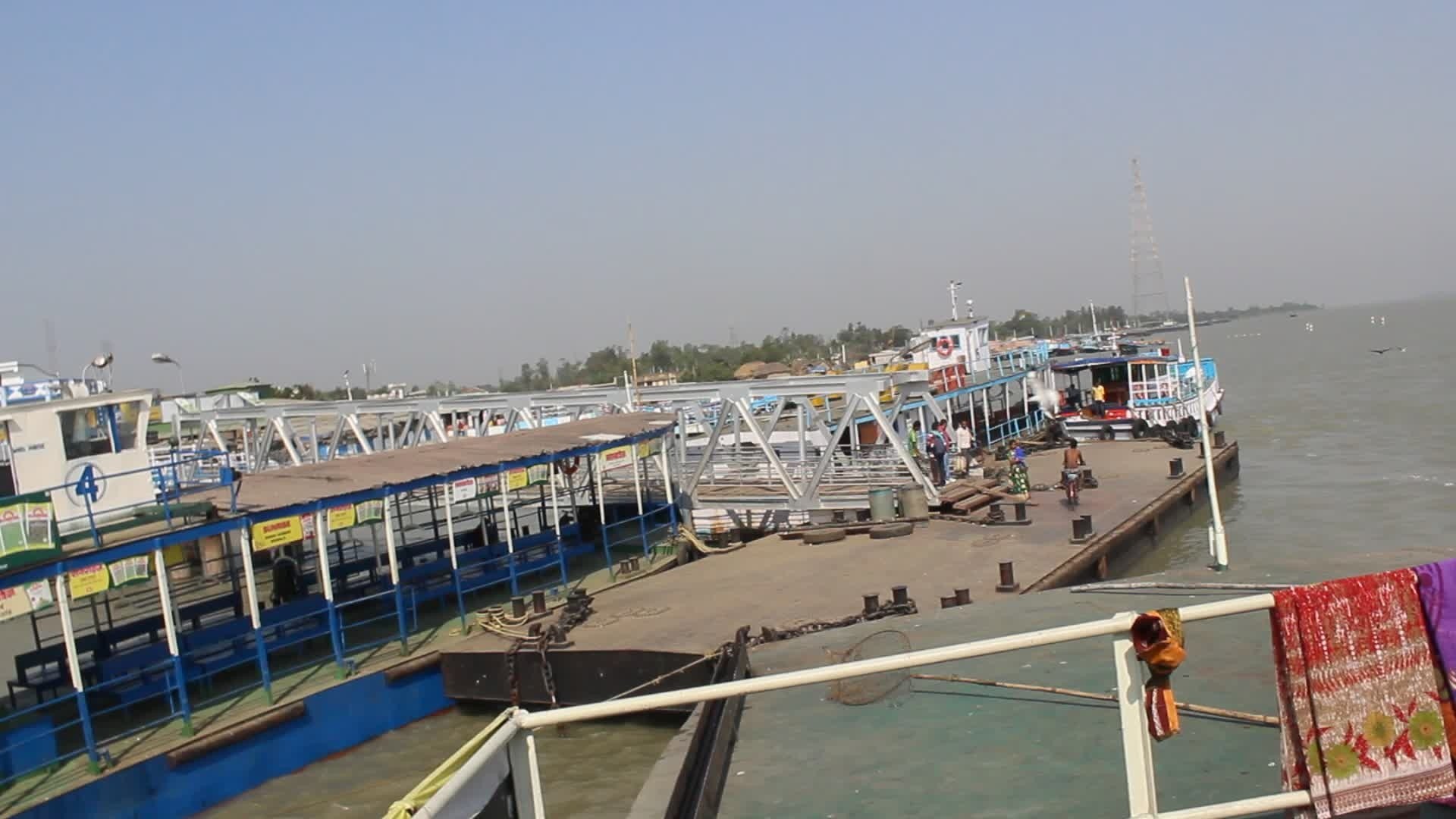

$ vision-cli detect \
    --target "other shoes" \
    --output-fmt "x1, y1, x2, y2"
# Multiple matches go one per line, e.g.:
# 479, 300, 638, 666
933, 482, 940, 486
953, 475, 958, 479
961, 474, 966, 479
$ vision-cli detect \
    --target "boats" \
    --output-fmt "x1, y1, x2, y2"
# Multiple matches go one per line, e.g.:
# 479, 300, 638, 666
380, 276, 1456, 819
0, 281, 1244, 819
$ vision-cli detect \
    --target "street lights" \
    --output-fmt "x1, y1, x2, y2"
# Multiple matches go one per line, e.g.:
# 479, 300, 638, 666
153, 354, 187, 397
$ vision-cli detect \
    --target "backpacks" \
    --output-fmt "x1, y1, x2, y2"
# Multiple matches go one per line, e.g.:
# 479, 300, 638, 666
933, 436, 945, 456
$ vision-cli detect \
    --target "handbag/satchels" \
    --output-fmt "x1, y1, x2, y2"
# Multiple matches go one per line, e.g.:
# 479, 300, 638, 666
953, 455, 967, 473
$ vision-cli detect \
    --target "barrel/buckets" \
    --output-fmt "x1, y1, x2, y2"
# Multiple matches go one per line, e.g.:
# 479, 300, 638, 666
897, 484, 903, 515
868, 486, 895, 521
901, 482, 929, 518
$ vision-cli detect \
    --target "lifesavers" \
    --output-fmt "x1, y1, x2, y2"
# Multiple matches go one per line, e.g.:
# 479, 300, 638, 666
868, 521, 913, 539
802, 527, 846, 544
1177, 423, 1188, 433
1150, 425, 1165, 437
1133, 418, 1148, 438
935, 338, 954, 357
1187, 415, 1198, 437
1166, 420, 1178, 438
1099, 425, 1115, 441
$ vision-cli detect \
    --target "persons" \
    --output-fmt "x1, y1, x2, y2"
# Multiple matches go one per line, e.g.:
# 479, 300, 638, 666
926, 419, 956, 486
1007, 440, 1031, 498
1068, 384, 1080, 406
1090, 381, 1105, 418
909, 420, 923, 467
1063, 439, 1085, 491
957, 421, 981, 472
